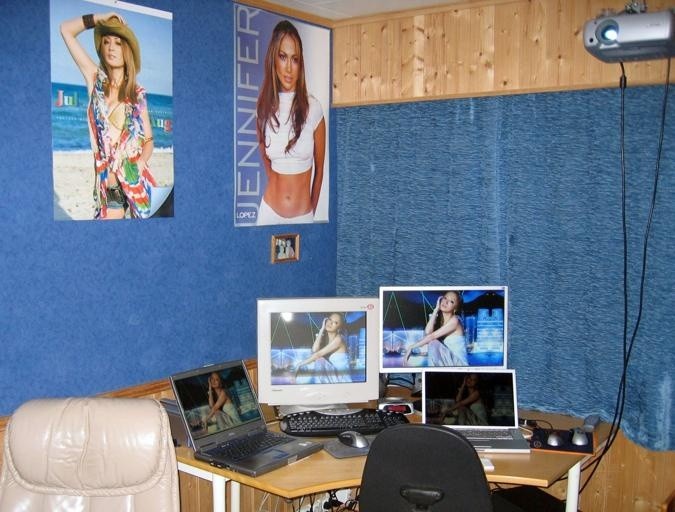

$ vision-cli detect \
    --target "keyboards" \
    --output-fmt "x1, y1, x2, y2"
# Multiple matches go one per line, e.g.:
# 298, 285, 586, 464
279, 408, 410, 437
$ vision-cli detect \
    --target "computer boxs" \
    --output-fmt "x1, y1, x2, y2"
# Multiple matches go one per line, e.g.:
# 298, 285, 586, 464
493, 486, 582, 511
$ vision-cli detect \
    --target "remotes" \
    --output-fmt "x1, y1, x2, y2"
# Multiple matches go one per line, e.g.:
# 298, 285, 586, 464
583, 415, 601, 430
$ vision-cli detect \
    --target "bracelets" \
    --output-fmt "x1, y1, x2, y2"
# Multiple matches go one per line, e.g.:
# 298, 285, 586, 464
459, 387, 462, 391
433, 309, 439, 316
319, 329, 324, 336
208, 390, 212, 394
82, 13, 96, 30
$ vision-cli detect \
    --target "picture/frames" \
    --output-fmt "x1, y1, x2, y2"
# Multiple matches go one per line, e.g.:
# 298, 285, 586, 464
269, 231, 302, 262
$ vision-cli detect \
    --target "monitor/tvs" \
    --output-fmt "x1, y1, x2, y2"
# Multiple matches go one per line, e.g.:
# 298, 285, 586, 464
255, 297, 379, 416
379, 285, 508, 416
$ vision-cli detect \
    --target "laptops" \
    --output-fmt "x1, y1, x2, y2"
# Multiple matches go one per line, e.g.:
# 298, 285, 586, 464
422, 370, 530, 453
168, 359, 323, 477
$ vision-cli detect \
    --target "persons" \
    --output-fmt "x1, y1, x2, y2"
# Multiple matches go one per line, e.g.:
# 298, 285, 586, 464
405, 290, 469, 367
60, 12, 158, 220
277, 245, 287, 259
285, 240, 295, 259
256, 21, 326, 226
440, 373, 489, 426
293, 313, 352, 383
202, 371, 243, 435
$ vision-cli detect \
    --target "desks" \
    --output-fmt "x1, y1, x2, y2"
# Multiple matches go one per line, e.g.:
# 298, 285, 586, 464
172, 406, 618, 512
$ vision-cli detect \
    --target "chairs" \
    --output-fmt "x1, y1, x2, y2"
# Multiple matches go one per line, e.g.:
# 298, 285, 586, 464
2, 395, 185, 512
356, 421, 495, 512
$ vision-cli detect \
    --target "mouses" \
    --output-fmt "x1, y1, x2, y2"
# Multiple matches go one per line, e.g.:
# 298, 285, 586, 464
570, 426, 588, 446
339, 430, 368, 448
542, 432, 565, 447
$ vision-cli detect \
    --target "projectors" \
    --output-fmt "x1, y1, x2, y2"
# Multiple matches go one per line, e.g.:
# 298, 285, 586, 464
583, 9, 675, 63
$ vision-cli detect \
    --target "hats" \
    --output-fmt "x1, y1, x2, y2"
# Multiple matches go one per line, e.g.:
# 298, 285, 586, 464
92, 15, 141, 70
